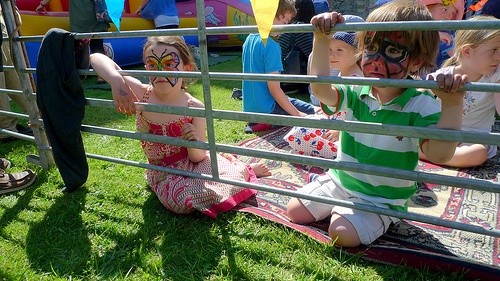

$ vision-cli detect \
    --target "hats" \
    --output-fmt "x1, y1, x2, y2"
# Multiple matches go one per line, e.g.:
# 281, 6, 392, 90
332, 15, 365, 48
421, 0, 464, 20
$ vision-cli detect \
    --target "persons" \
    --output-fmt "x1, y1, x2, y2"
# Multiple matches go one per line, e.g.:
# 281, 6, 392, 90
89, 35, 271, 217
0, 21, 37, 193
284, 1, 470, 249
69, 0, 500, 166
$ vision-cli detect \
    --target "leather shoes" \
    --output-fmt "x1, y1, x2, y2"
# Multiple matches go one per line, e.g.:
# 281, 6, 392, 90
410, 182, 438, 207
0, 124, 33, 143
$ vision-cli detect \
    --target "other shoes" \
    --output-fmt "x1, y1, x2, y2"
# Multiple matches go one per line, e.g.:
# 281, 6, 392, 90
98, 76, 106, 84
232, 88, 242, 100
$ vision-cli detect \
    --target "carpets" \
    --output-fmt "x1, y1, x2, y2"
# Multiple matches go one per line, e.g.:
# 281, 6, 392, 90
230, 120, 500, 281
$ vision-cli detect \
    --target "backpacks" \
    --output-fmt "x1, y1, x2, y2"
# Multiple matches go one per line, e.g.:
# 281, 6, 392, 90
313, 0, 329, 13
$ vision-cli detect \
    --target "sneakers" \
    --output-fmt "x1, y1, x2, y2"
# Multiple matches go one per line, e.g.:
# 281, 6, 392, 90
281, 84, 299, 95
0, 158, 36, 194
